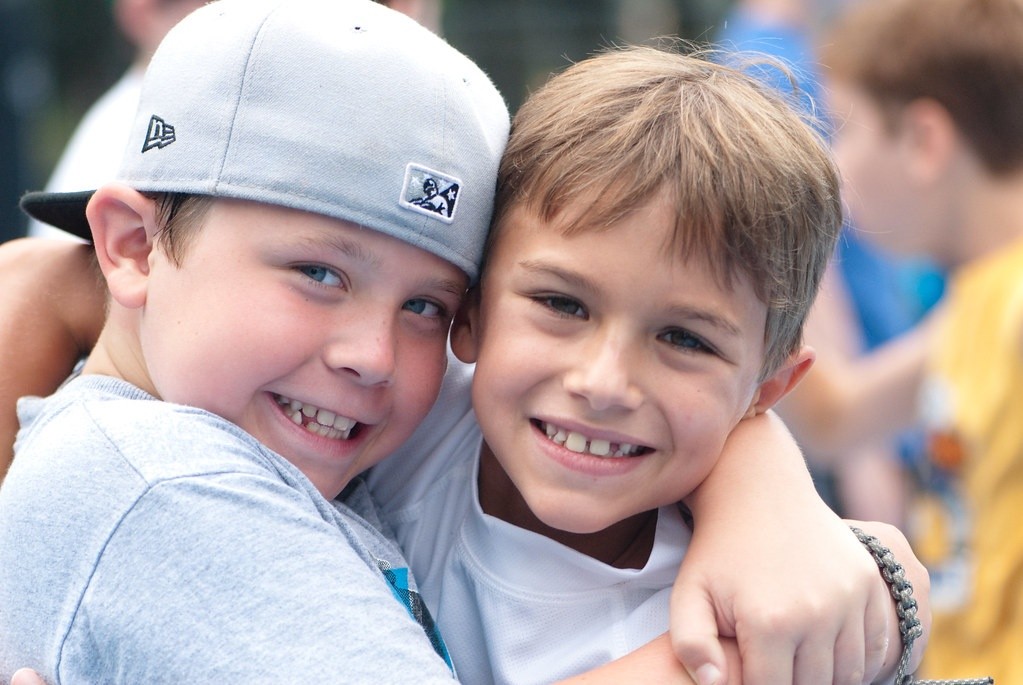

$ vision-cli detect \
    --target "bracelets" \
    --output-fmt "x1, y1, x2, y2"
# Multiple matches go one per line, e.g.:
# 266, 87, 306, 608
849, 526, 994, 685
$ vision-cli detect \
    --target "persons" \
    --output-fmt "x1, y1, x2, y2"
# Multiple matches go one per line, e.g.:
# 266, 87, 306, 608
0, 0, 1023, 685
0, 0, 935, 685
0, 37, 845, 684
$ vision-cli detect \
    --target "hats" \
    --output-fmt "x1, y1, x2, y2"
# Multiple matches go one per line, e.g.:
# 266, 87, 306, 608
19, 0, 513, 288
